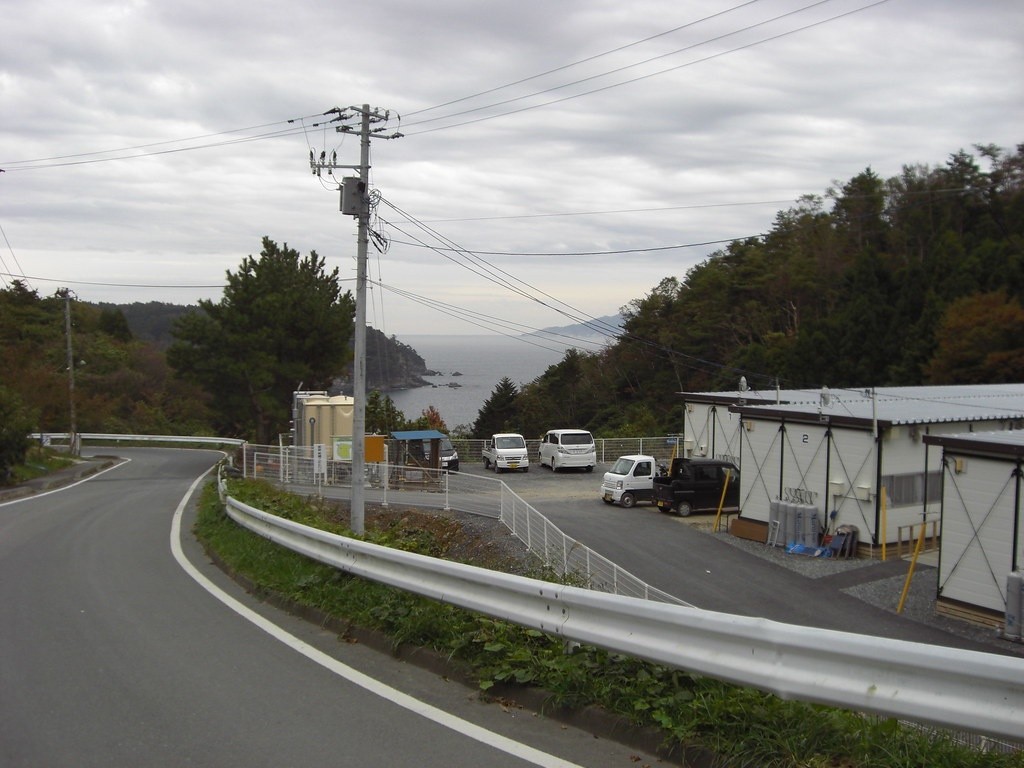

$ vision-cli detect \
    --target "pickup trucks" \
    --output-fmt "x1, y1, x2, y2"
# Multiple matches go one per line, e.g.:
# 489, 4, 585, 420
653, 456, 740, 516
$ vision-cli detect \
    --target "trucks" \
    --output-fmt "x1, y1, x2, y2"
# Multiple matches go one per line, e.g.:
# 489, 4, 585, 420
482, 433, 530, 473
600, 455, 668, 508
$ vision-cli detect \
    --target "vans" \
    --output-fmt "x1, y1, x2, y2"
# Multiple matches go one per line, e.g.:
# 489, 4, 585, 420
538, 429, 597, 473
422, 439, 459, 472
